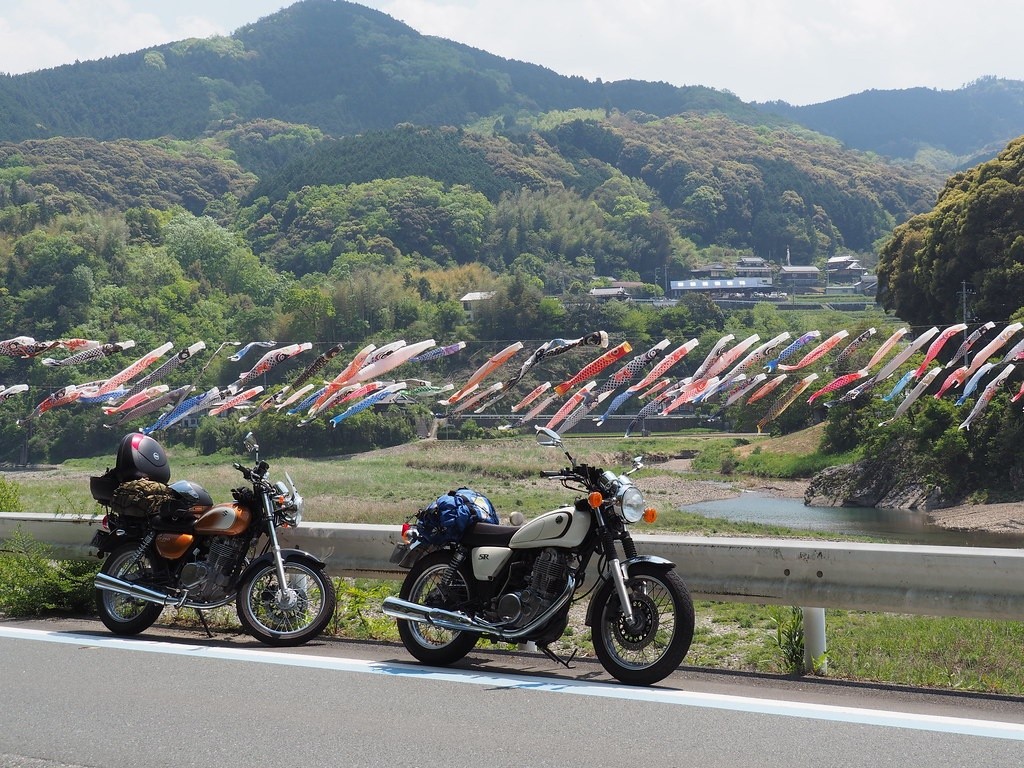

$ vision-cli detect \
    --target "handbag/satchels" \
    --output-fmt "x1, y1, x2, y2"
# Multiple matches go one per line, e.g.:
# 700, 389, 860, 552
416, 486, 499, 548
109, 482, 174, 517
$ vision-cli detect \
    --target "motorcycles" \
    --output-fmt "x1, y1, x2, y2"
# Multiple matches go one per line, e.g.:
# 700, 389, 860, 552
380, 428, 697, 686
88, 429, 338, 648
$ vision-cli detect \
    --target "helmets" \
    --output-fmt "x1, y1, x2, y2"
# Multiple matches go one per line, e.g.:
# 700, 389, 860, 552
159, 479, 213, 528
